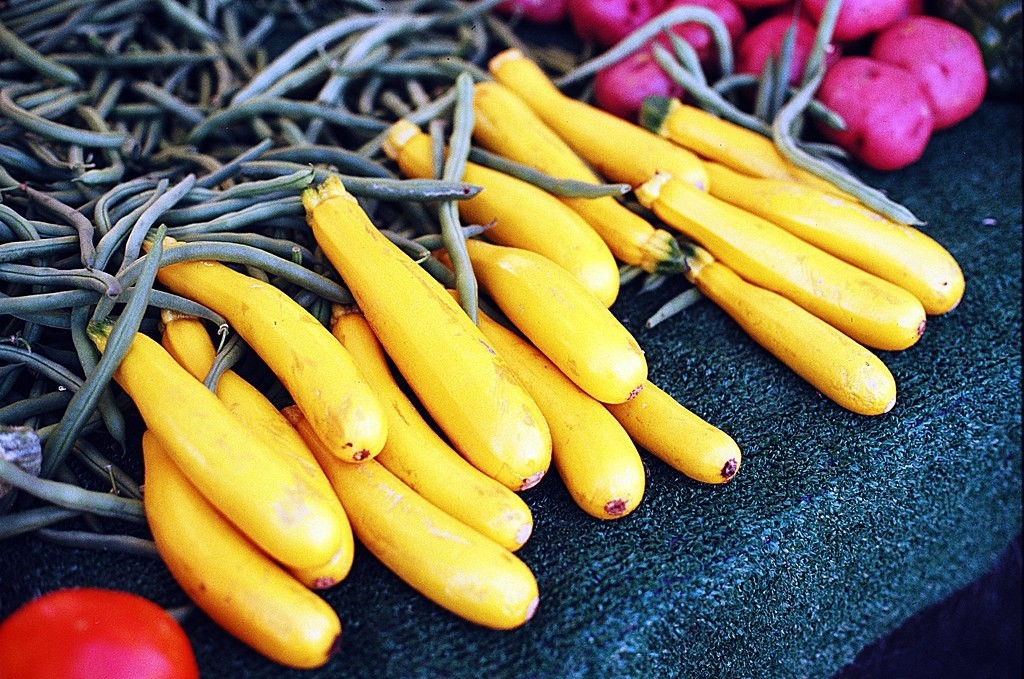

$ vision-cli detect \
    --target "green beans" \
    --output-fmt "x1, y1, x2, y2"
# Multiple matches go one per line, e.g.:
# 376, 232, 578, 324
0, 0, 919, 560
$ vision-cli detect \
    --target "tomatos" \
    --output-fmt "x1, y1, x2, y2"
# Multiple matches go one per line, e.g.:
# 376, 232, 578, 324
0, 589, 200, 679
